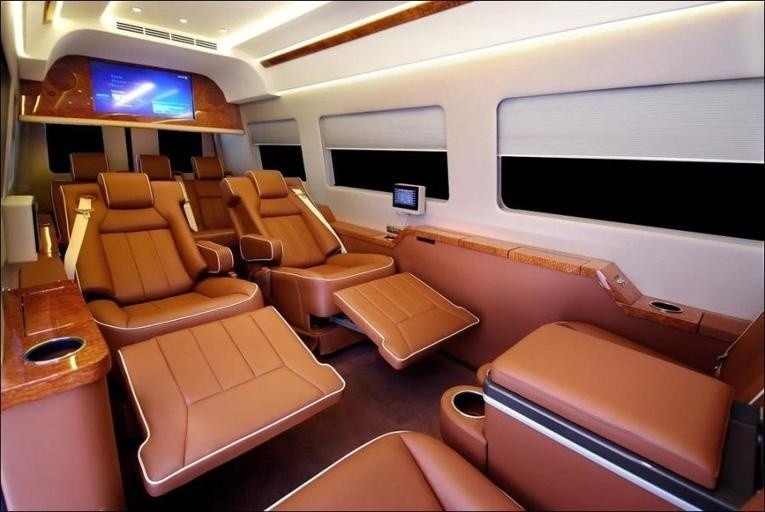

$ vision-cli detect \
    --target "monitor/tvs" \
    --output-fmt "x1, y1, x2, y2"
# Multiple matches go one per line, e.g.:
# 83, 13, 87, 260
390, 182, 426, 216
89, 61, 195, 120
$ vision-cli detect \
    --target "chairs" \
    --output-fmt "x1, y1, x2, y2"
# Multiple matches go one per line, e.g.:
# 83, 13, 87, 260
49, 153, 764, 510
